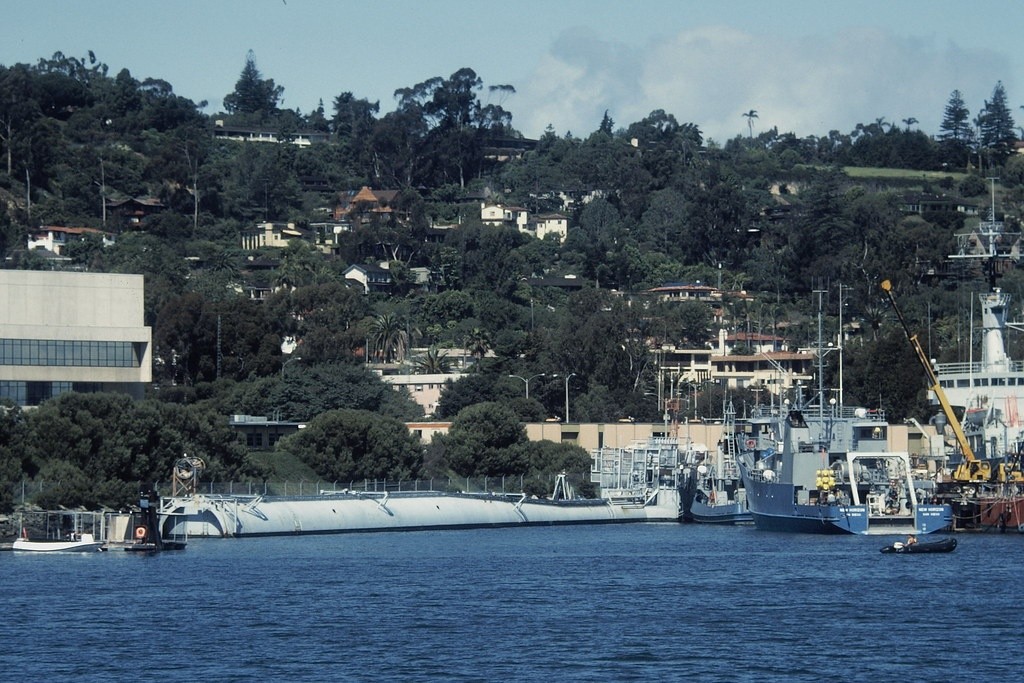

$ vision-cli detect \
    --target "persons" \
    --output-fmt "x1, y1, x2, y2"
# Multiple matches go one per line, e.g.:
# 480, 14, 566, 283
900, 534, 914, 547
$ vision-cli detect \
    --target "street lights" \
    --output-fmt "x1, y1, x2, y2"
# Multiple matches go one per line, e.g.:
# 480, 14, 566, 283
509, 374, 547, 399
553, 373, 577, 422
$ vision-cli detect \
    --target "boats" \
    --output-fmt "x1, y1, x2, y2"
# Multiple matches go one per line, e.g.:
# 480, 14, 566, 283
879, 529, 969, 555
11, 521, 109, 555
685, 411, 911, 539
121, 511, 189, 553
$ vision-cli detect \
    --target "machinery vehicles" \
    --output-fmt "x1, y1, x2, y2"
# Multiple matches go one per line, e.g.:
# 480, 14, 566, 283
878, 279, 1024, 485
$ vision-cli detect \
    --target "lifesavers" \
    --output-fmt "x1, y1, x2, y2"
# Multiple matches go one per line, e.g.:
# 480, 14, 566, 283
137, 527, 146, 538
747, 439, 756, 448
